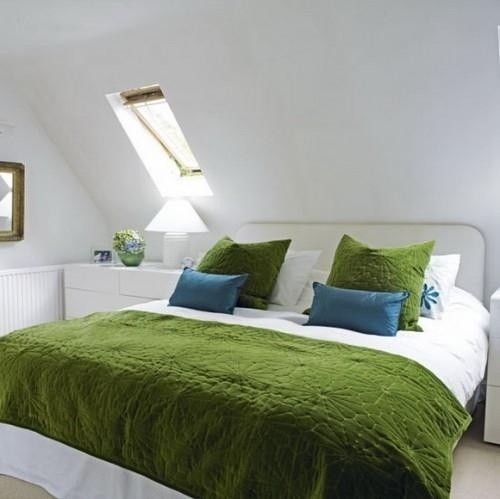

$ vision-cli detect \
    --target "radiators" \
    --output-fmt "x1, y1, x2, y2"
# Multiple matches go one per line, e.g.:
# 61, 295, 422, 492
0, 264, 64, 337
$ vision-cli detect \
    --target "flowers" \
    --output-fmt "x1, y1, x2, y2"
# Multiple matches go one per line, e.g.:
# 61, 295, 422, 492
112, 229, 146, 256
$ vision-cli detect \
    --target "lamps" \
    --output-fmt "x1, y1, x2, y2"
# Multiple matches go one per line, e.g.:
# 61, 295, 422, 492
144, 199, 209, 269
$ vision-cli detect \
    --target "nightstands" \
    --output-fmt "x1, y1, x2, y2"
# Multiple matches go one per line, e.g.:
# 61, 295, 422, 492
64, 262, 197, 321
483, 287, 500, 445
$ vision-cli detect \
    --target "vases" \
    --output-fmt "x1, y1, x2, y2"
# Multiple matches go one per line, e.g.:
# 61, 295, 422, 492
118, 251, 145, 266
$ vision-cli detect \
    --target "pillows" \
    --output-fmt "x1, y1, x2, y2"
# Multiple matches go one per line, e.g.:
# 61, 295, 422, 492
302, 282, 411, 336
292, 269, 330, 313
419, 253, 460, 322
302, 233, 436, 332
167, 266, 250, 315
268, 247, 322, 306
195, 234, 292, 311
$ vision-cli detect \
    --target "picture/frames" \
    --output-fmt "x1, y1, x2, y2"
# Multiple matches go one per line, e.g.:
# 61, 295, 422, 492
91, 248, 114, 264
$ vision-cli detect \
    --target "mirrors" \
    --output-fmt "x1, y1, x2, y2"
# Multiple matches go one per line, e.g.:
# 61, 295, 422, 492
0, 161, 24, 241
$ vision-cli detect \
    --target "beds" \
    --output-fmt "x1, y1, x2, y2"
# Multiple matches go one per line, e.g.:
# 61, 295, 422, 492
1, 223, 487, 498
